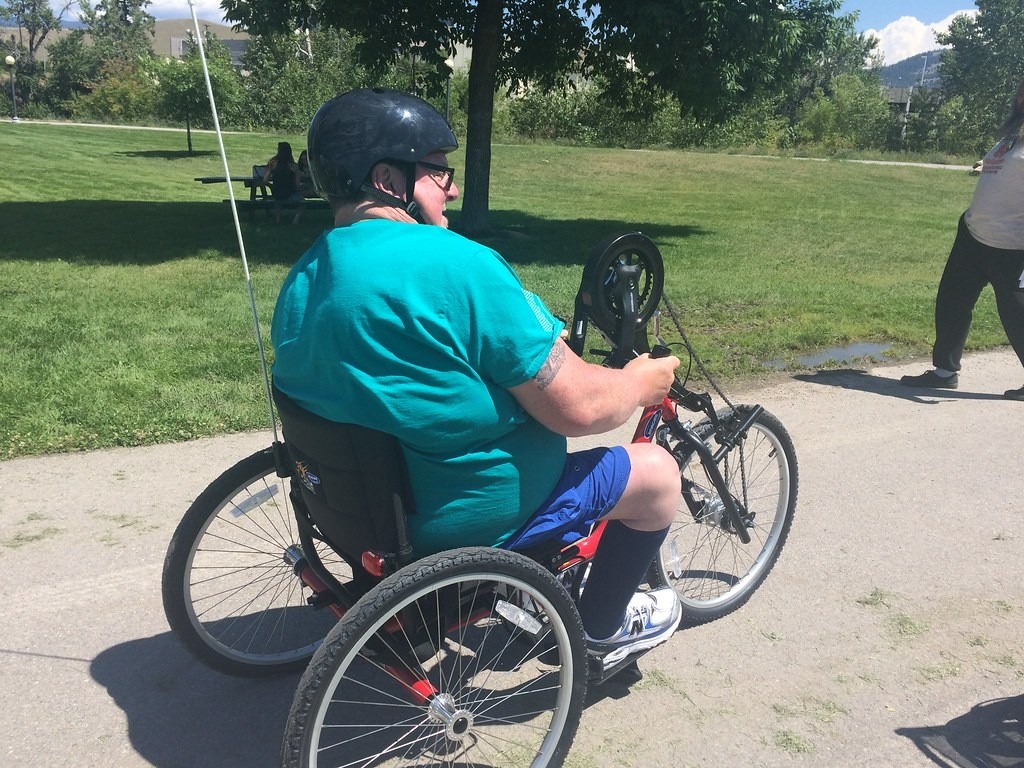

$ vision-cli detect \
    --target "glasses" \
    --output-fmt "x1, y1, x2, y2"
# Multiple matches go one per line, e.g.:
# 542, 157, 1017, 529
417, 161, 455, 191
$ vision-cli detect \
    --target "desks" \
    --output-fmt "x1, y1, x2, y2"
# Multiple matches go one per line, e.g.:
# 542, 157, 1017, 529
243, 180, 321, 218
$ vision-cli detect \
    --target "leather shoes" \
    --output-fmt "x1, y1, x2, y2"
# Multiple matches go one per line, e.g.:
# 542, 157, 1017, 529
1004, 385, 1024, 401
901, 370, 959, 389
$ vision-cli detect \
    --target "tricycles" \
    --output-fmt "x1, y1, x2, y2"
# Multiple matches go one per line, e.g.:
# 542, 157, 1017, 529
158, 227, 800, 768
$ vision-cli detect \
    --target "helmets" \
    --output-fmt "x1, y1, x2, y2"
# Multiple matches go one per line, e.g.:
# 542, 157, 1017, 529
307, 87, 459, 203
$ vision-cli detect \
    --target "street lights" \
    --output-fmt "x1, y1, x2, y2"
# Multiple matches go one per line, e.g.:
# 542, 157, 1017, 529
920, 55, 928, 87
6, 55, 19, 121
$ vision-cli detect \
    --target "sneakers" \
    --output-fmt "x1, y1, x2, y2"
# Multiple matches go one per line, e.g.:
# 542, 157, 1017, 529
583, 588, 682, 672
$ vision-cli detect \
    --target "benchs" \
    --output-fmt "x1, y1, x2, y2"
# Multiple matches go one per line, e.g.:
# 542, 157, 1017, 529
222, 198, 331, 223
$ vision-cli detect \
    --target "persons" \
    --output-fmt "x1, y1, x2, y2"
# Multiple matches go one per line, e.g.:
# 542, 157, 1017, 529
262, 141, 315, 229
271, 87, 683, 675
900, 76, 1024, 402
